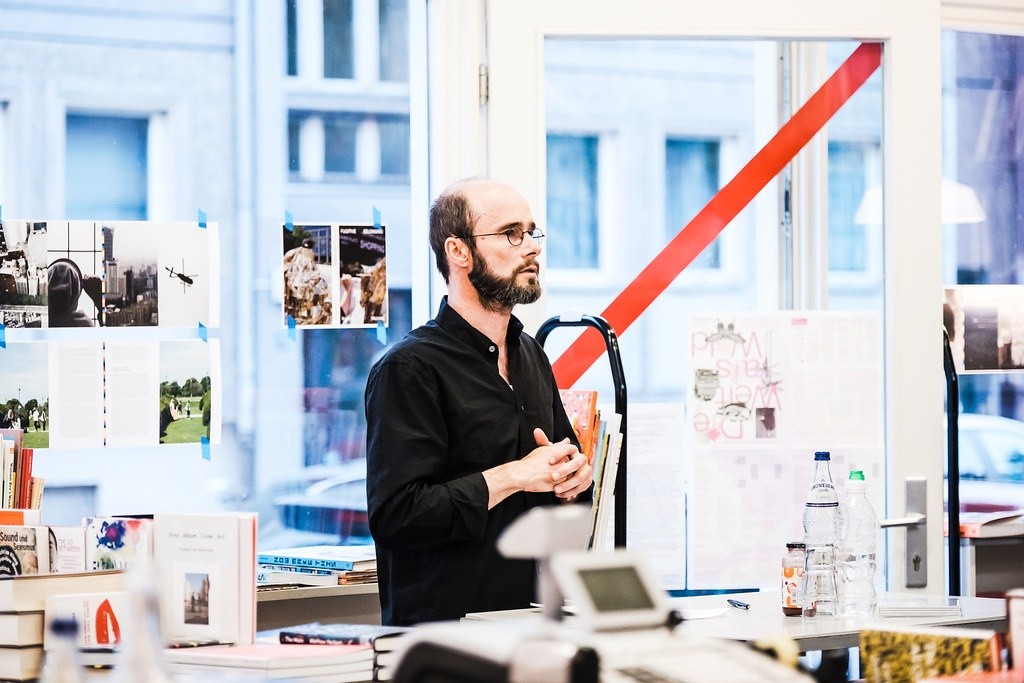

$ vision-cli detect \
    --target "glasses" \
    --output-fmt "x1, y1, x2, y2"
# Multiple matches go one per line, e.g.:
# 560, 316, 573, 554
455, 226, 546, 249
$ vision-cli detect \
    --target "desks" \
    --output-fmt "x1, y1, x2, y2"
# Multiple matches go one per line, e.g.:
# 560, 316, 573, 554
256, 583, 381, 633
960, 538, 1024, 615
674, 590, 1014, 683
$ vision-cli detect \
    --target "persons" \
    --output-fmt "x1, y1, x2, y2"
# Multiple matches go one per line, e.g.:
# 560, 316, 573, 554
170, 399, 190, 419
6, 405, 47, 433
49, 528, 60, 572
364, 178, 592, 625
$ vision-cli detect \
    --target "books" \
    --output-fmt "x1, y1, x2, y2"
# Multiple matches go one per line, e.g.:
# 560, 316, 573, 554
877, 593, 962, 618
0, 511, 412, 683
0, 428, 45, 511
558, 389, 624, 551
460, 609, 577, 624
944, 510, 1024, 538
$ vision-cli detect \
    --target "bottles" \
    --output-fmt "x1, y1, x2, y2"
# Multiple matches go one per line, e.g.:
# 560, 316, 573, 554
837, 470, 876, 618
781, 541, 808, 618
800, 450, 837, 616
